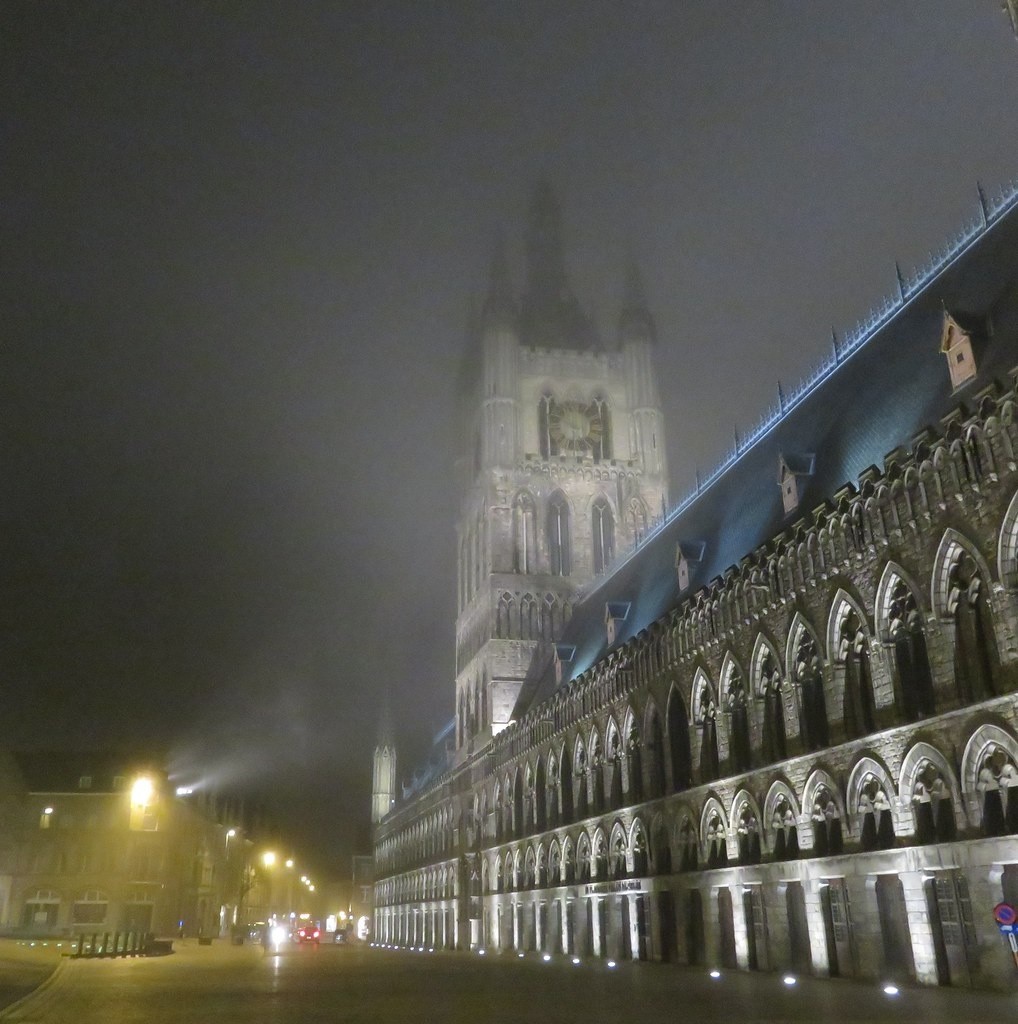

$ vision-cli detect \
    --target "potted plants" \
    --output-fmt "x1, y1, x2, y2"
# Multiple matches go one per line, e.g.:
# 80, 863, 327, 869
198, 931, 213, 945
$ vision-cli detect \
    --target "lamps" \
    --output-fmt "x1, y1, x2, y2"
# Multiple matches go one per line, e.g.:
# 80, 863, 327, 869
42, 804, 55, 816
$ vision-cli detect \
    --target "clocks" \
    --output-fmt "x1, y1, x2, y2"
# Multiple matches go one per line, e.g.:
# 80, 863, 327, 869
550, 400, 602, 451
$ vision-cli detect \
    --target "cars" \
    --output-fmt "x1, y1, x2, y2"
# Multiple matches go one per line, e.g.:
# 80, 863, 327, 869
300, 926, 322, 944
334, 929, 347, 943
